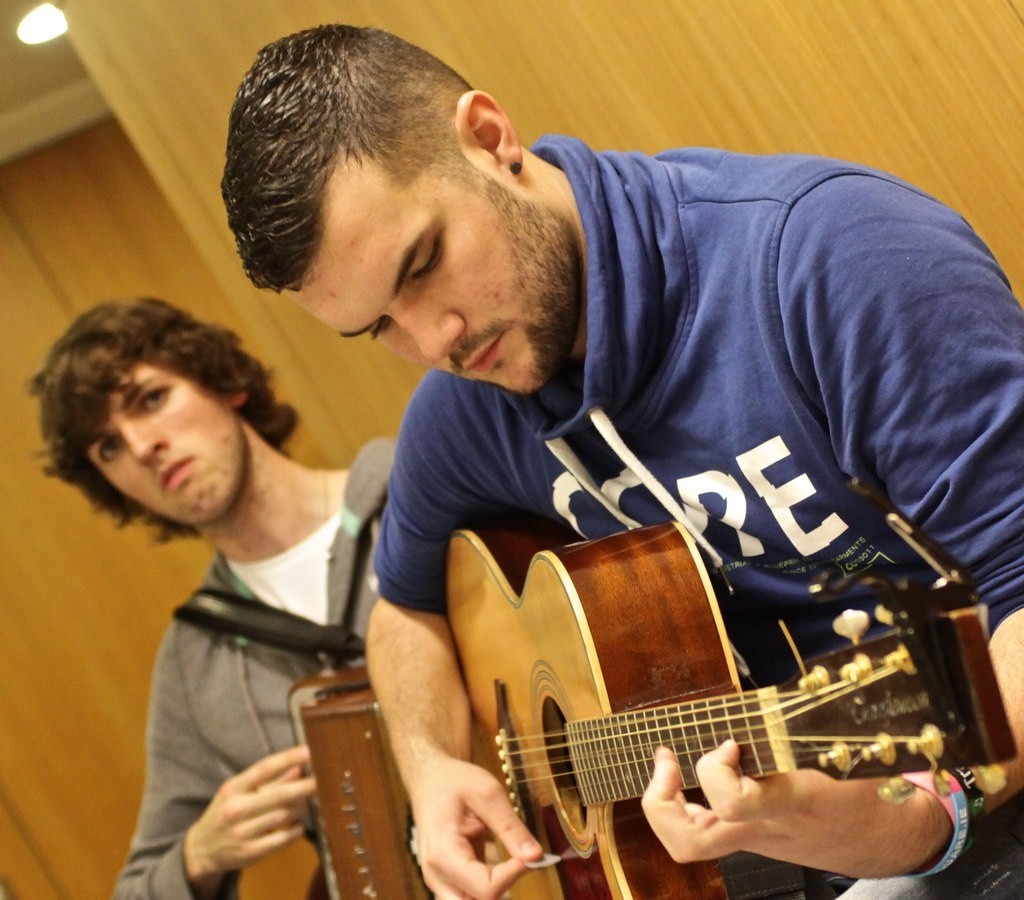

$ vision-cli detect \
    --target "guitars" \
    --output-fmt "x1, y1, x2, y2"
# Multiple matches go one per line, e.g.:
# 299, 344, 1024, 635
441, 514, 1019, 900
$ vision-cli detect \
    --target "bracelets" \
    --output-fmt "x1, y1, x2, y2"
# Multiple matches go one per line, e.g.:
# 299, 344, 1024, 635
898, 765, 986, 878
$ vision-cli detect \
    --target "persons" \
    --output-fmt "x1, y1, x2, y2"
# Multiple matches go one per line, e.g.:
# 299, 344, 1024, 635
20, 295, 398, 900
222, 22, 1024, 900
